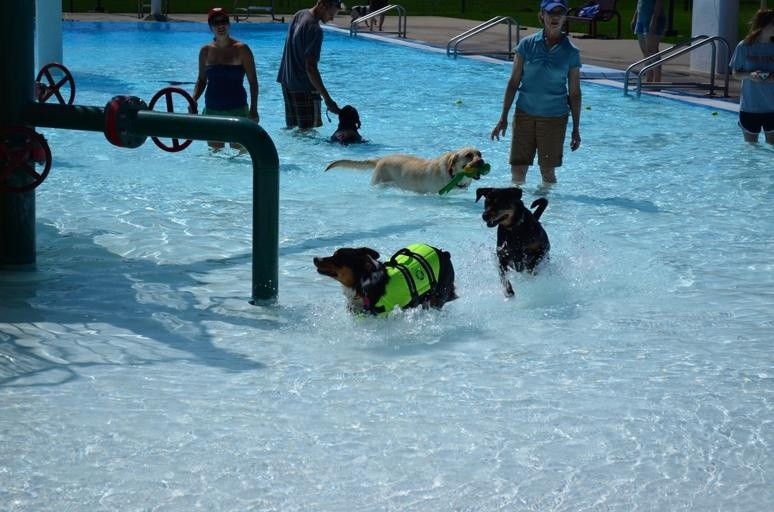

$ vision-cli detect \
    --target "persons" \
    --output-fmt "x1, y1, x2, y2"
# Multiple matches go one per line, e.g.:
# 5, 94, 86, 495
369, 0, 388, 31
187, 8, 259, 149
728, 8, 774, 146
491, 0, 582, 182
277, 0, 343, 130
630, 0, 665, 91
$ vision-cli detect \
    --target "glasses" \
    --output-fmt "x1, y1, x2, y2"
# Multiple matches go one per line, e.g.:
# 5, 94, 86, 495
211, 18, 228, 26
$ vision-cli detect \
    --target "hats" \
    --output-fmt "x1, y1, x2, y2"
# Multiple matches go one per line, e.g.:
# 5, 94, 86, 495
208, 7, 228, 17
540, 0, 569, 13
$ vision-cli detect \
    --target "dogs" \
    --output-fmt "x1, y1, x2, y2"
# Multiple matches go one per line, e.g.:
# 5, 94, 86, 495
324, 146, 491, 198
474, 185, 552, 297
313, 242, 457, 315
329, 104, 368, 143
